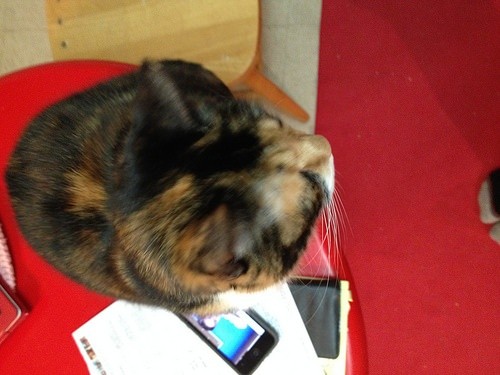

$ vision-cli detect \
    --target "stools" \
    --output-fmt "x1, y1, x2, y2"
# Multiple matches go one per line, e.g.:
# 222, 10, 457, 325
44, 0, 310, 124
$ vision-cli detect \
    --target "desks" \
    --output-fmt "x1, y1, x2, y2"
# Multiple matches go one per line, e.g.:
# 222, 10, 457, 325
0, 59, 373, 375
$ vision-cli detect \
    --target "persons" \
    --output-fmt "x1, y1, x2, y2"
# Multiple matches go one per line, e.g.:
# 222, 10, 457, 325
198, 313, 246, 333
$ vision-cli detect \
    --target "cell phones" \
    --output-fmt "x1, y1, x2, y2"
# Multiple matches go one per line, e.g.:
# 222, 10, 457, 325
174, 308, 279, 375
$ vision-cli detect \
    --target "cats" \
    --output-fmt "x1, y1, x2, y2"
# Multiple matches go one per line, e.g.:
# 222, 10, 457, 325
4, 57, 353, 321
